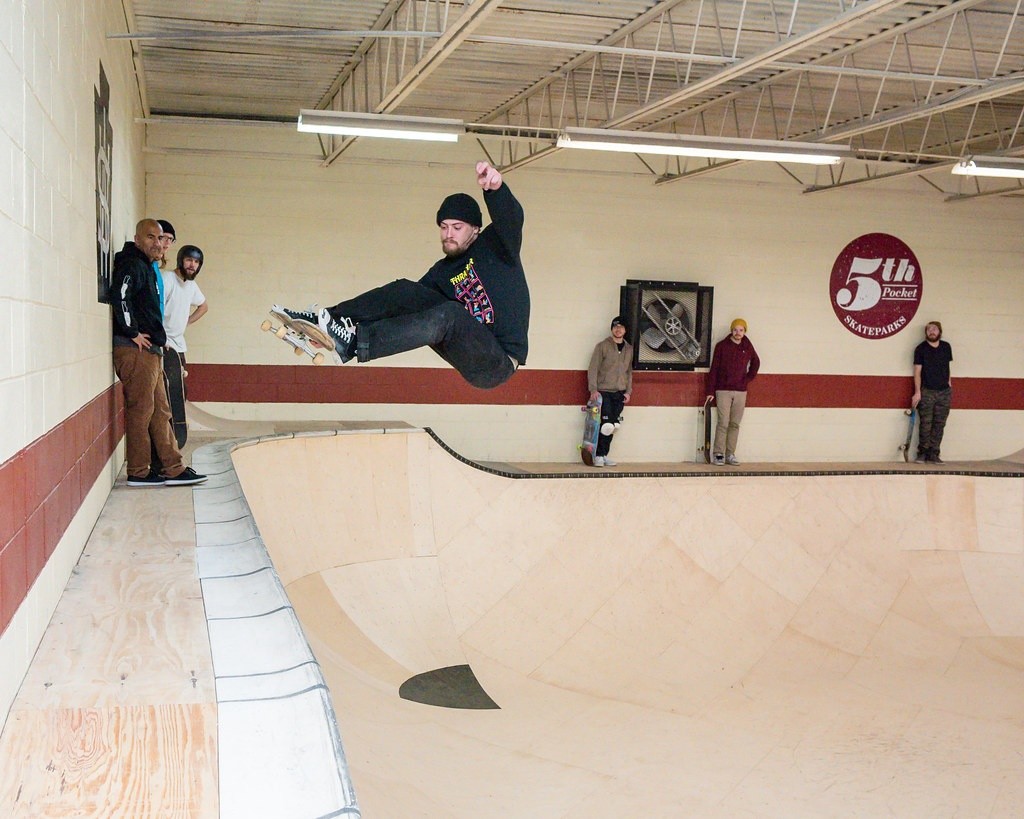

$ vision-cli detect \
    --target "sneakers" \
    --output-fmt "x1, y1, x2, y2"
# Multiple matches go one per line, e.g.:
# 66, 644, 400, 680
713, 452, 724, 466
271, 303, 319, 326
603, 456, 617, 465
594, 456, 604, 466
126, 469, 164, 487
318, 308, 356, 365
726, 454, 740, 466
165, 467, 207, 486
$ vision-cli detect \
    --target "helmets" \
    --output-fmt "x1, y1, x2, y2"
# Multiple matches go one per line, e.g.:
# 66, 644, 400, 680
177, 245, 204, 281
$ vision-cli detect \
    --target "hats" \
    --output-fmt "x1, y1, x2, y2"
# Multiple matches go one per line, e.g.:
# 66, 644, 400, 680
157, 219, 176, 243
610, 316, 628, 331
436, 193, 482, 228
730, 319, 747, 332
929, 321, 942, 332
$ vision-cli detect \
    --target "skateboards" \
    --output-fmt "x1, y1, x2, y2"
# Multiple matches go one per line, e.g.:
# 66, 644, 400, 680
261, 308, 335, 365
898, 399, 920, 462
696, 396, 712, 464
577, 391, 602, 465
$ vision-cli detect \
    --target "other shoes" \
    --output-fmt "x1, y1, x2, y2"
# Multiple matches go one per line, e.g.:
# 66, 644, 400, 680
915, 452, 925, 463
926, 456, 945, 465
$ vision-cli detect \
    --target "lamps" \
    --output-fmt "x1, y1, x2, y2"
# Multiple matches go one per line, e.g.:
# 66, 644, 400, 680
297, 109, 465, 142
951, 155, 1024, 179
557, 126, 851, 165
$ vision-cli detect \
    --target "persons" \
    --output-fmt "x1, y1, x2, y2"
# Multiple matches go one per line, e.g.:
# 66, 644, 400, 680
587, 316, 634, 466
146, 220, 176, 472
271, 161, 531, 389
160, 245, 208, 400
109, 218, 208, 486
706, 319, 760, 466
912, 321, 953, 465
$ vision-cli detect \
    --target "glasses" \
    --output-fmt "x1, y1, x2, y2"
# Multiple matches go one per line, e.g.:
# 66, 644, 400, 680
162, 236, 176, 244
